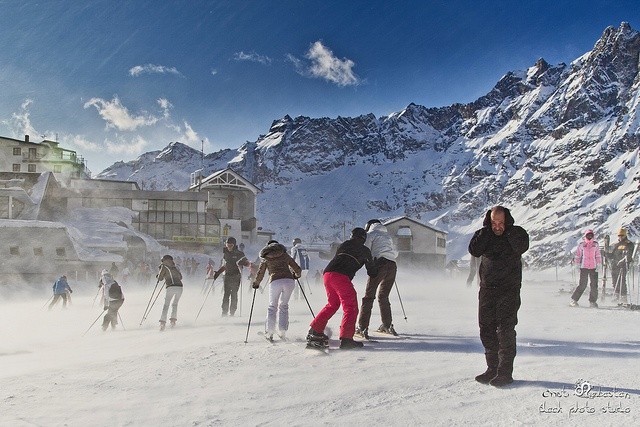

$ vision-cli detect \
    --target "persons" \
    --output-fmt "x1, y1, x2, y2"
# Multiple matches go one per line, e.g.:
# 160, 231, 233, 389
354, 219, 408, 341
156, 255, 184, 332
312, 270, 322, 284
105, 249, 159, 284
48, 274, 73, 310
468, 206, 529, 387
305, 227, 389, 352
214, 237, 249, 317
568, 229, 602, 308
289, 237, 312, 301
98, 271, 125, 332
252, 239, 302, 343
175, 251, 214, 281
248, 258, 263, 293
603, 227, 634, 301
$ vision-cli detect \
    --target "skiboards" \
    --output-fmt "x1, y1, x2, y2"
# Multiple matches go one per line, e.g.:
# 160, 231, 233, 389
356, 326, 410, 343
258, 325, 289, 344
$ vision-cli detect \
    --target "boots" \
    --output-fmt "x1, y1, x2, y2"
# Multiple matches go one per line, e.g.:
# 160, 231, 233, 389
489, 356, 513, 388
475, 353, 498, 385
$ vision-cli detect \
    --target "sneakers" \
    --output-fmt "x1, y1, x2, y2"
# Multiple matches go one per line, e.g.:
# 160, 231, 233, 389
356, 327, 369, 335
340, 339, 363, 350
378, 322, 391, 330
307, 333, 329, 342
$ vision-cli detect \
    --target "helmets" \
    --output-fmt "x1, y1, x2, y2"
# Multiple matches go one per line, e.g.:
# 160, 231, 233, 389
366, 219, 381, 231
293, 238, 301, 246
351, 227, 367, 241
161, 255, 173, 262
227, 237, 236, 245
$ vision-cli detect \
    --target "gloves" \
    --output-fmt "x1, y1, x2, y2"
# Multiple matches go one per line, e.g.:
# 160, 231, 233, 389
213, 271, 220, 280
483, 210, 492, 230
253, 282, 259, 290
505, 208, 514, 227
292, 272, 300, 279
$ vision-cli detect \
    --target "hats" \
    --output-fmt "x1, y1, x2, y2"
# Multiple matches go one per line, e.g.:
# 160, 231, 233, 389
617, 228, 627, 236
